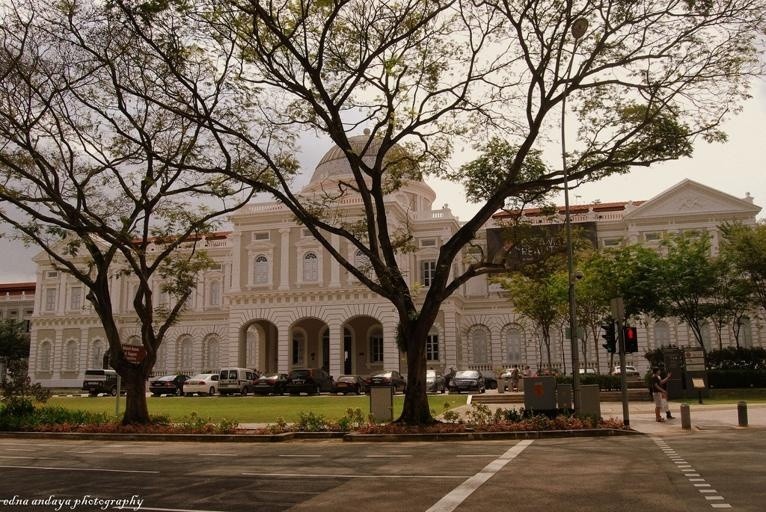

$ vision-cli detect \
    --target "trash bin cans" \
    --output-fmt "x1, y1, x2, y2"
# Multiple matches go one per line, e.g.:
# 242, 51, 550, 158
498, 380, 504, 392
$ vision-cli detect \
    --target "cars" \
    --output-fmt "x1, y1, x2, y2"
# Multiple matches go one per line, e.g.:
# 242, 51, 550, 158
334, 375, 369, 395
183, 374, 219, 395
613, 365, 638, 374
572, 368, 596, 374
149, 373, 190, 396
426, 369, 445, 394
368, 371, 407, 394
448, 368, 514, 394
267, 389, 269, 390
253, 373, 290, 395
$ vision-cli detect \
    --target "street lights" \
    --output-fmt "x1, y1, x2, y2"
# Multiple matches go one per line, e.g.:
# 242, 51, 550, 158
561, 13, 590, 419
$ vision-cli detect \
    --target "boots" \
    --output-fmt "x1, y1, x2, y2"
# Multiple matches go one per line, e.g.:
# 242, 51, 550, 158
666, 410, 675, 419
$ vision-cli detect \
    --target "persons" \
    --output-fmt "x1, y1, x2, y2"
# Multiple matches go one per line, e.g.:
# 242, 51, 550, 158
447, 366, 454, 377
519, 364, 532, 378
652, 373, 676, 420
650, 367, 671, 422
511, 364, 518, 388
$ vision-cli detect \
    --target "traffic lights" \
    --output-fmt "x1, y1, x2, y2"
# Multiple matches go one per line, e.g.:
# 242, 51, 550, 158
625, 326, 638, 352
600, 321, 617, 352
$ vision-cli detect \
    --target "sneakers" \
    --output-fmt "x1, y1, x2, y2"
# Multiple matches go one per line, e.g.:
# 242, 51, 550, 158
656, 416, 666, 422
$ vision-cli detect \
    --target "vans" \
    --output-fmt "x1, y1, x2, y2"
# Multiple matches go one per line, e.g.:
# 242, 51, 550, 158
287, 368, 335, 397
83, 368, 126, 395
219, 367, 262, 396
